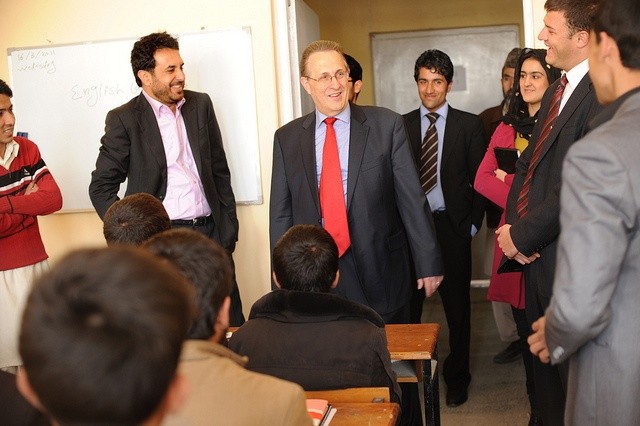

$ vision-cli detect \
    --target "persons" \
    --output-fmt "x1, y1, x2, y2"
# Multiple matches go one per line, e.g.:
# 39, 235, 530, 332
143, 226, 316, 425
228, 222, 405, 426
494, 0, 606, 425
268, 39, 449, 425
402, 48, 486, 409
88, 30, 245, 327
472, 47, 562, 425
0, 78, 61, 374
478, 60, 536, 365
102, 191, 171, 246
343, 54, 364, 105
13, 244, 195, 426
526, 0, 639, 426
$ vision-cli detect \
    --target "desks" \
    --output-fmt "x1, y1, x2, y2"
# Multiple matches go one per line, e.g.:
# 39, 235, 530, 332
300, 399, 398, 426
225, 320, 439, 424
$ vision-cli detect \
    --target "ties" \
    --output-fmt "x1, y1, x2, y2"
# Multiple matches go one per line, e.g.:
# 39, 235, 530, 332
419, 113, 440, 195
516, 74, 569, 222
320, 118, 352, 258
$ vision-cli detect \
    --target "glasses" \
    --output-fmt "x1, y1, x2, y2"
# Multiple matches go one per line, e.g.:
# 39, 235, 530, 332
304, 69, 348, 85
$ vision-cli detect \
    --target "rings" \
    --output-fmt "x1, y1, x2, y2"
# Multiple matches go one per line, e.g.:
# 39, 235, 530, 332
435, 281, 443, 285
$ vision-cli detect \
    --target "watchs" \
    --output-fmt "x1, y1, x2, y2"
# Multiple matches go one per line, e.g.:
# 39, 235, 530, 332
225, 331, 233, 343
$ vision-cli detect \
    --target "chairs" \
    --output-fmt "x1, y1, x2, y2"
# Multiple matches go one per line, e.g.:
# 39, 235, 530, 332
303, 387, 392, 403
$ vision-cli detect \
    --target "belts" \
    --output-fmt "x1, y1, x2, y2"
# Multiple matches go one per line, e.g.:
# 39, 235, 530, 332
172, 216, 213, 226
431, 209, 448, 219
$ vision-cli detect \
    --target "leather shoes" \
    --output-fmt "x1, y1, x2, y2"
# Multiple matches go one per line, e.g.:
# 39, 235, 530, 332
493, 340, 521, 363
446, 370, 471, 407
